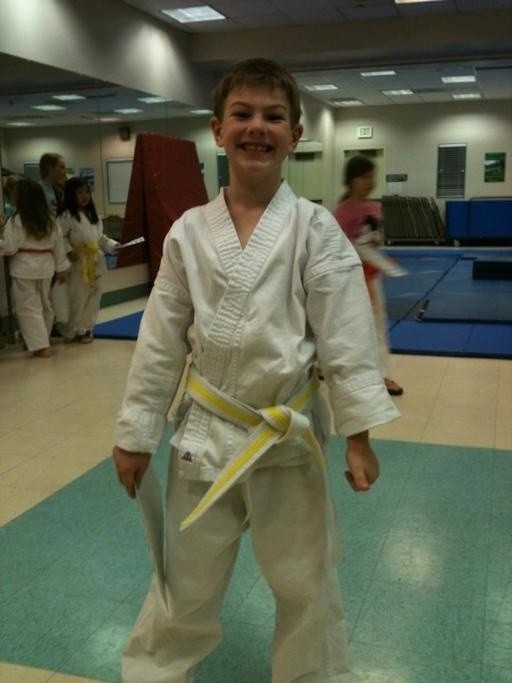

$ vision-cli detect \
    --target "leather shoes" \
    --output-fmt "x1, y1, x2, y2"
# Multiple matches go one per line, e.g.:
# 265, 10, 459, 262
385, 379, 403, 394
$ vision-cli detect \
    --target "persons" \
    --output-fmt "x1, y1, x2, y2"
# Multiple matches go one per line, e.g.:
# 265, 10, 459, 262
328, 153, 403, 396
54, 175, 124, 343
0, 177, 71, 357
107, 54, 402, 682
3, 173, 30, 206
36, 151, 68, 218
